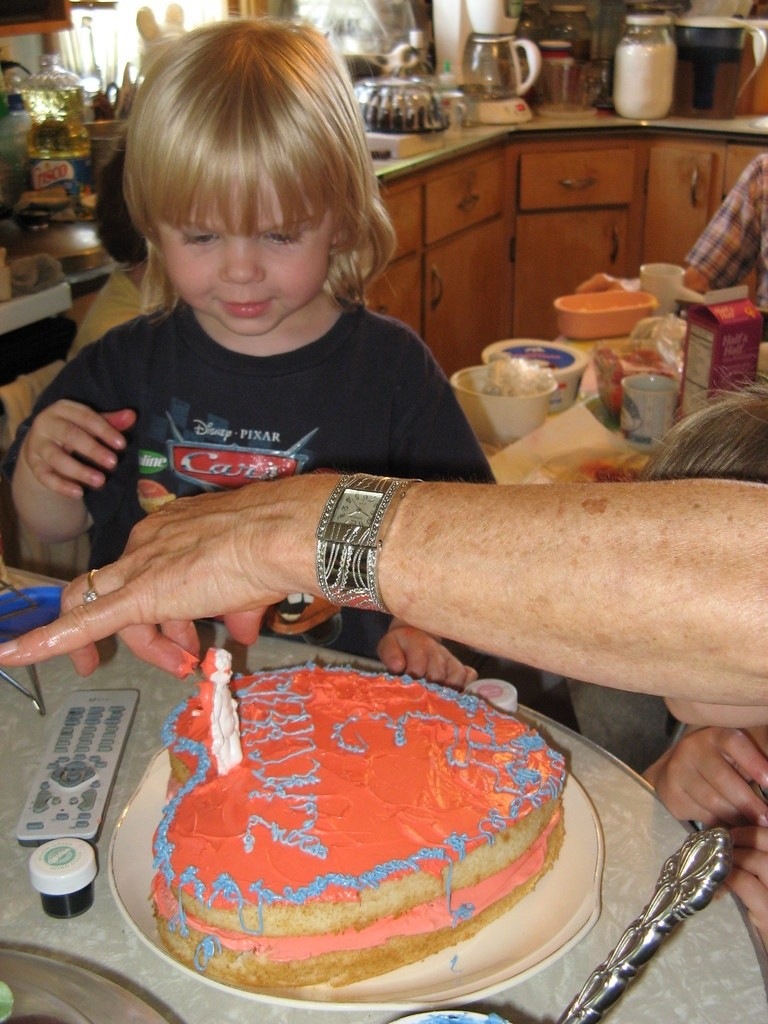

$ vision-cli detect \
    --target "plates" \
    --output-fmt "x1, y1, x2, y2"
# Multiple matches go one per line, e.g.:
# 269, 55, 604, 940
388, 1011, 513, 1024
0, 949, 169, 1024
107, 746, 607, 1013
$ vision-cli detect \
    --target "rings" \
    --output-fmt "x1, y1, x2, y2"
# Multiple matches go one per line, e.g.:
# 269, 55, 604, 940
81, 567, 101, 606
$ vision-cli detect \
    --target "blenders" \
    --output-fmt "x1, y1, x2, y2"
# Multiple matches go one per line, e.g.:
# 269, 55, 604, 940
460, 0, 542, 126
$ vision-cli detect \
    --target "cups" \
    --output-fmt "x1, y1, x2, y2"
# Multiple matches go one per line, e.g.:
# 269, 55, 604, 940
449, 366, 558, 456
640, 263, 686, 318
620, 374, 680, 455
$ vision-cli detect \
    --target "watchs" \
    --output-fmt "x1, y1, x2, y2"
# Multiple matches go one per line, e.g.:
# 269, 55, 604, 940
316, 473, 419, 616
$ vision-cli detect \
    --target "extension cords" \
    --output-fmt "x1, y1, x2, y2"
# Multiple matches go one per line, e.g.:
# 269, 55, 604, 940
0, 43, 15, 92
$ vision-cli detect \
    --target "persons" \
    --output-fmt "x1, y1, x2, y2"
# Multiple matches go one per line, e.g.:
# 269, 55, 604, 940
0, 473, 768, 706
683, 152, 768, 308
561, 380, 768, 948
3, 16, 497, 690
63, 145, 170, 363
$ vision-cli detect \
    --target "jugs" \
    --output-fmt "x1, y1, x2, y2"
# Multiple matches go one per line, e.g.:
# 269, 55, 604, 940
673, 16, 768, 120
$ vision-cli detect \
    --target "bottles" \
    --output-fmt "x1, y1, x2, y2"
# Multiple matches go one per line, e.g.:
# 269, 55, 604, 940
546, 4, 591, 64
18, 53, 94, 222
29, 837, 98, 919
612, 15, 678, 120
515, 0, 548, 56
399, 30, 431, 76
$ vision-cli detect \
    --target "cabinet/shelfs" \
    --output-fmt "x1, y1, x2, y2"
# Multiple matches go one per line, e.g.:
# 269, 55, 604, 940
368, 141, 767, 378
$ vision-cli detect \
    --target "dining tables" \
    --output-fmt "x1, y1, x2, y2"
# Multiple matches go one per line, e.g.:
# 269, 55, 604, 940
0, 568, 768, 1024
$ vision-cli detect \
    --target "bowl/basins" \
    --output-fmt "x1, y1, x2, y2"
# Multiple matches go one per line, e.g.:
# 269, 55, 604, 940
480, 337, 592, 415
553, 290, 660, 340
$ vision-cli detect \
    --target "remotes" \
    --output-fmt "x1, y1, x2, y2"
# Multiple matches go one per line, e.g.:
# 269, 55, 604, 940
15, 689, 140, 846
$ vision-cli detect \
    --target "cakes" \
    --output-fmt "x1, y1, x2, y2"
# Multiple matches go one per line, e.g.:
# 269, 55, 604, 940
148, 649, 567, 990
